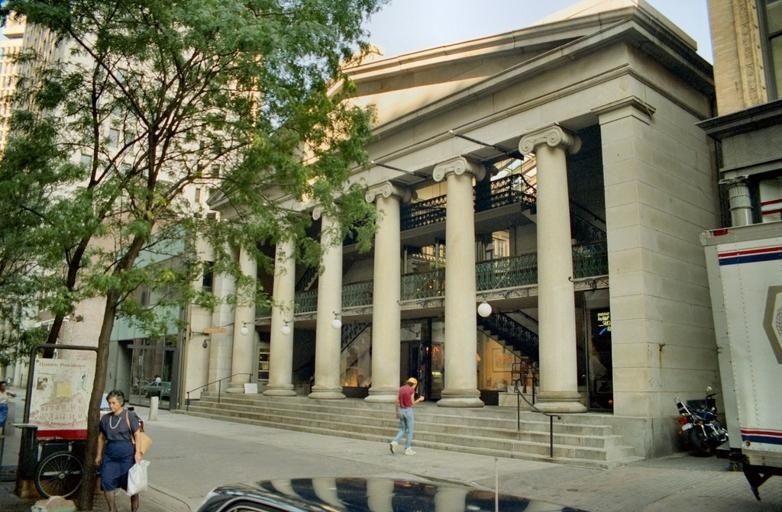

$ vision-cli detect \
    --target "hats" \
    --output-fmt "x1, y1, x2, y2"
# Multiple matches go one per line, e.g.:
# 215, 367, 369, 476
408, 378, 417, 384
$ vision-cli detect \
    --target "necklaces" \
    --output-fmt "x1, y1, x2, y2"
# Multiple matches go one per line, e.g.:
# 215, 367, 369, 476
108, 416, 121, 430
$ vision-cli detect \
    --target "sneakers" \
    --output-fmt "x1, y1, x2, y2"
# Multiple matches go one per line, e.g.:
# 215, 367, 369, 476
391, 442, 397, 453
404, 448, 415, 455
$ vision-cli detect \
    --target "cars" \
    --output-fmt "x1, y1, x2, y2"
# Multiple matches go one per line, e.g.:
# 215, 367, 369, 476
101, 391, 133, 422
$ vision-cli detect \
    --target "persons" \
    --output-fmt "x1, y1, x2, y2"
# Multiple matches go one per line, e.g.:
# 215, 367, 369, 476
93, 388, 142, 512
0, 381, 17, 435
390, 377, 425, 455
155, 374, 161, 385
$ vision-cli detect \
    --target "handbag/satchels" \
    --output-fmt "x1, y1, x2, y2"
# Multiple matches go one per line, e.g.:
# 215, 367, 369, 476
140, 432, 151, 455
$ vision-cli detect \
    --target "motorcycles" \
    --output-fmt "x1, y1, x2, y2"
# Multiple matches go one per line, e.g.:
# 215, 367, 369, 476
673, 385, 729, 457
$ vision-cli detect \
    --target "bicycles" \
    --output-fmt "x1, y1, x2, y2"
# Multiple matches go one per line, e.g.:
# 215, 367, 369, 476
36, 436, 101, 498
